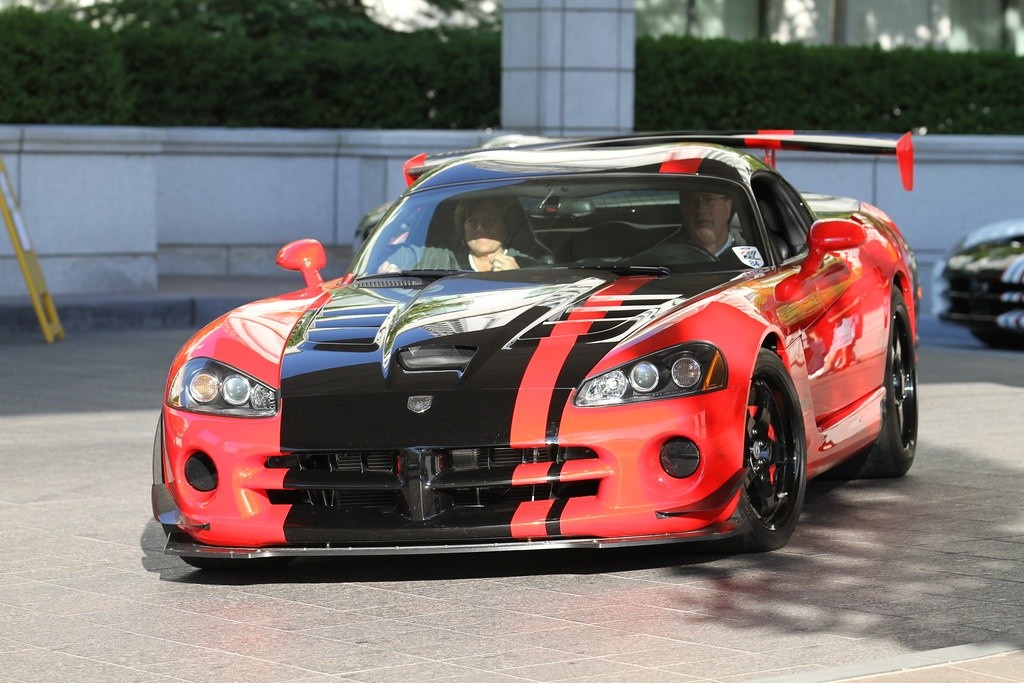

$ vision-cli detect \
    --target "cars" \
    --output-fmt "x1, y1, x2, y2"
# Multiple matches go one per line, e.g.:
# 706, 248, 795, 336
931, 219, 1024, 347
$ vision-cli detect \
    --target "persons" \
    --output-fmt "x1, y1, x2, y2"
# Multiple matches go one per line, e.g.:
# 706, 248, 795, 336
378, 197, 544, 275
654, 182, 752, 265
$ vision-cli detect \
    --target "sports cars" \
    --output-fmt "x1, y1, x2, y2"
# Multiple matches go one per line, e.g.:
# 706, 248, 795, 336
151, 131, 920, 574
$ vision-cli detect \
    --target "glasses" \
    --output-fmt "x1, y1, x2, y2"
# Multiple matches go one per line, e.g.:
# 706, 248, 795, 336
680, 194, 725, 208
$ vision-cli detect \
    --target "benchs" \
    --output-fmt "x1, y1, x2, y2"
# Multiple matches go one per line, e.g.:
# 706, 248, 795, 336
532, 220, 682, 265
